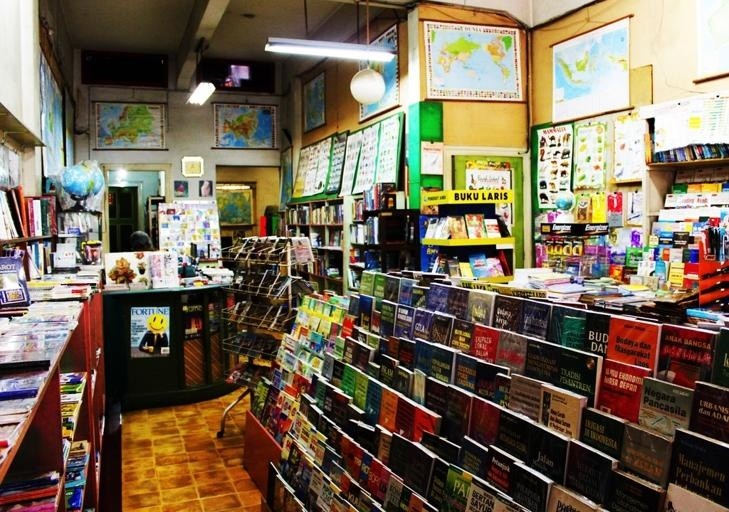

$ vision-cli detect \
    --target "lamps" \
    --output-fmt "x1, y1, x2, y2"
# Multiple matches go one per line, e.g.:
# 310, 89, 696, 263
261, 0, 395, 63
183, 31, 215, 109
348, 0, 388, 105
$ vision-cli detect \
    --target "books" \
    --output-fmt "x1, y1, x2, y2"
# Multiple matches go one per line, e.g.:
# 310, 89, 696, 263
250, 271, 729, 511
286, 182, 404, 309
1, 186, 104, 510
229, 237, 316, 387
633, 141, 729, 290
427, 214, 509, 278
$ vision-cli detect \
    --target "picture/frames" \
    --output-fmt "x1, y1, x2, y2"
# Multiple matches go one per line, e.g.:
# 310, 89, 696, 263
180, 156, 205, 179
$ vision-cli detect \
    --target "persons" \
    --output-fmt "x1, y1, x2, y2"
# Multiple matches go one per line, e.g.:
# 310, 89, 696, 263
139, 330, 168, 351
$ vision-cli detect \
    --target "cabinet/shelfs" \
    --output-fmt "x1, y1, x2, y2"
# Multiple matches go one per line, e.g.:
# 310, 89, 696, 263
2, 193, 108, 511
221, 192, 728, 512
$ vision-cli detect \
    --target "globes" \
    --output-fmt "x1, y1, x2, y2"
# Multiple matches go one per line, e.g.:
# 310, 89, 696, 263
61, 159, 104, 211
